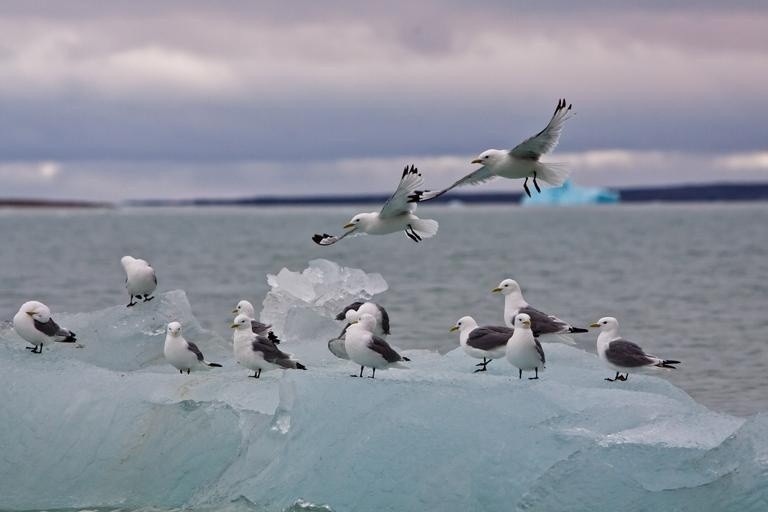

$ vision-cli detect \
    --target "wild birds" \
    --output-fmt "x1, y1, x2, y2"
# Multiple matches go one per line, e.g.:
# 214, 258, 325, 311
12, 298, 75, 355
228, 298, 307, 379
312, 161, 438, 247
450, 316, 541, 373
118, 255, 160, 308
502, 312, 548, 382
490, 277, 590, 339
163, 321, 223, 375
406, 97, 578, 205
326, 299, 413, 380
588, 315, 682, 382
